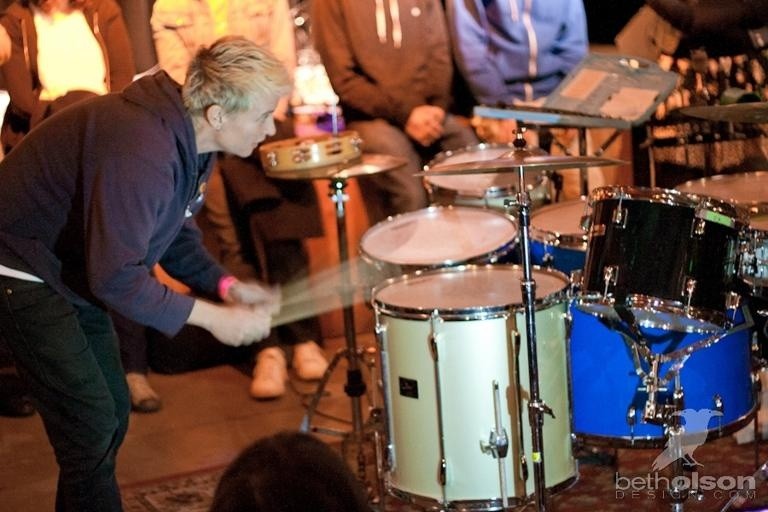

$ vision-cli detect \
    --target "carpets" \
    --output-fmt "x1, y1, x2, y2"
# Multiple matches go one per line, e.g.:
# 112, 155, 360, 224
119, 437, 768, 512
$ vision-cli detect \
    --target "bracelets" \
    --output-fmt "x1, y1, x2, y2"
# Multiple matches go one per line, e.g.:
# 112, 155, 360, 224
219, 276, 240, 300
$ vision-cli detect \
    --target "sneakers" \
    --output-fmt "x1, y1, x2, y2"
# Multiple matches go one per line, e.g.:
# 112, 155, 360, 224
124, 373, 162, 413
292, 339, 330, 380
249, 346, 288, 400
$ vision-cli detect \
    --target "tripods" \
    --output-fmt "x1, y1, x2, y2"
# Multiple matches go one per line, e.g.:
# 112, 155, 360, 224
299, 181, 381, 481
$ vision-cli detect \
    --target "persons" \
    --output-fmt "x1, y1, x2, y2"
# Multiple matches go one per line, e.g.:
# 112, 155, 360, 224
0, 36, 300, 512
307, 0, 480, 215
2, 0, 162, 415
1, 0, 142, 157
211, 425, 371, 511
446, 0, 605, 202
150, 2, 329, 402
683, 44, 727, 104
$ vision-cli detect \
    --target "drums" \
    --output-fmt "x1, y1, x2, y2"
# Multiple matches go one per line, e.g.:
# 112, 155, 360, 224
356, 171, 768, 507
421, 142, 562, 216
260, 130, 362, 175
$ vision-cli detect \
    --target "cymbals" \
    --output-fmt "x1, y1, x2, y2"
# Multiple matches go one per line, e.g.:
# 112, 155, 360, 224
678, 102, 768, 124
266, 154, 408, 181
412, 155, 627, 179
